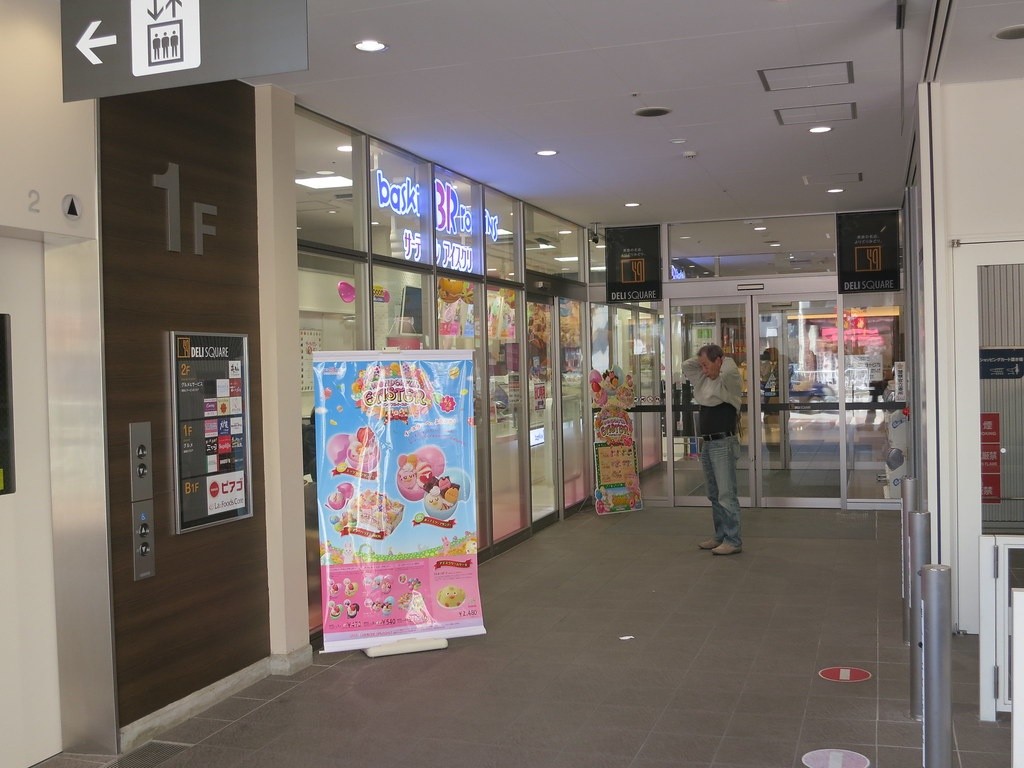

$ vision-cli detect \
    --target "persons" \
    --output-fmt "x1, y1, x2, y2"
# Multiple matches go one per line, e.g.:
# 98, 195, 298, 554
302, 407, 315, 483
681, 344, 744, 554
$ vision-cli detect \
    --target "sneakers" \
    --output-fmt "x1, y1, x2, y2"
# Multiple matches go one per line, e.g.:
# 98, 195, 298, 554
711, 543, 742, 554
698, 538, 722, 548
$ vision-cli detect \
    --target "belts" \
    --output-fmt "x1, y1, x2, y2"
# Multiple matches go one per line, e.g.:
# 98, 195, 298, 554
703, 431, 736, 441
